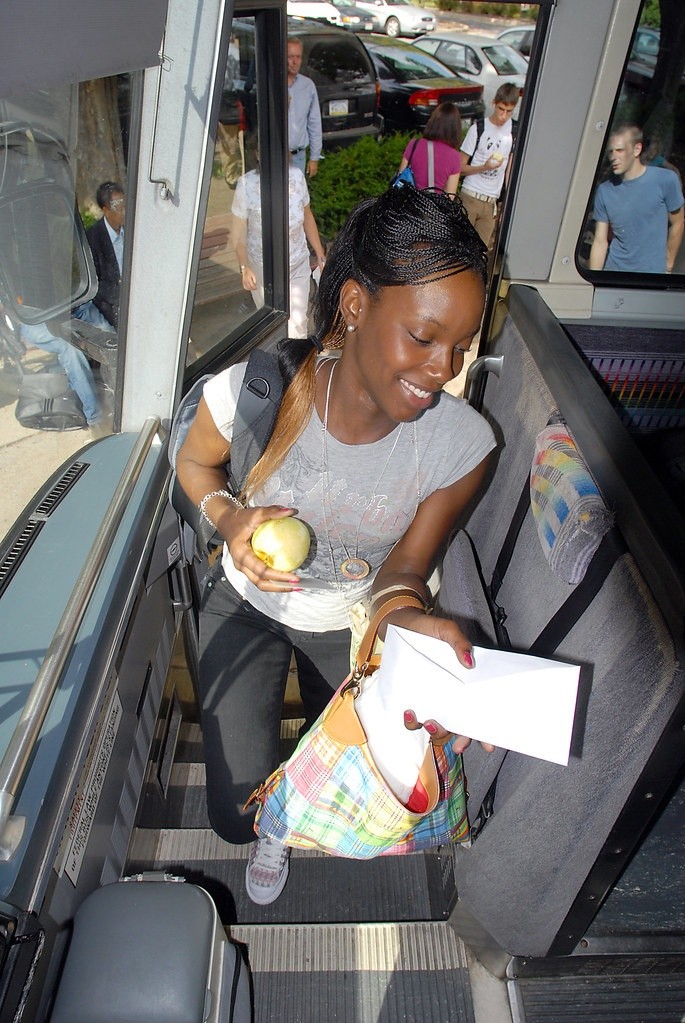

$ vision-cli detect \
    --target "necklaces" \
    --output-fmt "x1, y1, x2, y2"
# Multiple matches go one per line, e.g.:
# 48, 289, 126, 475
315, 356, 421, 638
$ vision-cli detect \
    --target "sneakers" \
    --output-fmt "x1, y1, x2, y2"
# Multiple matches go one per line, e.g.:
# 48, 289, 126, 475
244, 837, 293, 906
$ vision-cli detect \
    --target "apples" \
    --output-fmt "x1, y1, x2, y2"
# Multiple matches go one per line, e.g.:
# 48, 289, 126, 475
493, 153, 503, 161
252, 516, 310, 572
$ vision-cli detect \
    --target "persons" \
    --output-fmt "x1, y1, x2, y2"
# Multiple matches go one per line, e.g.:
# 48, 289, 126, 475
285, 35, 325, 176
0, 181, 126, 442
230, 136, 328, 339
458, 82, 521, 249
399, 102, 465, 200
176, 180, 497, 904
587, 123, 685, 275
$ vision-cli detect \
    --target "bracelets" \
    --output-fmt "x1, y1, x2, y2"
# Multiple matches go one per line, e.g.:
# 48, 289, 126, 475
199, 490, 243, 529
240, 265, 248, 272
667, 269, 672, 272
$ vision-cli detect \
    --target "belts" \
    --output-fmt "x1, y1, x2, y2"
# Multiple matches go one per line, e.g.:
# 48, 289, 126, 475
291, 149, 302, 154
461, 188, 497, 219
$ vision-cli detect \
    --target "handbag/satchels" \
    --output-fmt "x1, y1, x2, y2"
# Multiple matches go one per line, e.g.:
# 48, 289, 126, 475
167, 350, 284, 558
388, 139, 416, 191
242, 595, 472, 861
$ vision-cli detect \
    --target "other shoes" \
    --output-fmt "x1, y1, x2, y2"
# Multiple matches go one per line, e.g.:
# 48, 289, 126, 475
89, 423, 112, 440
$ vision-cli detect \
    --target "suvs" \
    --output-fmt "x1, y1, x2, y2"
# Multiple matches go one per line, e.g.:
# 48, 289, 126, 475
223, 12, 386, 148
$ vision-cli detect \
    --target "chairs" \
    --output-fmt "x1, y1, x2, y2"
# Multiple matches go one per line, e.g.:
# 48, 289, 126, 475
436, 47, 447, 63
448, 49, 459, 64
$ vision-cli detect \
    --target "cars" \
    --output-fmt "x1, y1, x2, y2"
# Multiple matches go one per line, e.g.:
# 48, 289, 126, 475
287, 0, 341, 26
355, 32, 485, 141
495, 26, 537, 62
345, 0, 439, 39
410, 32, 530, 121
624, 27, 661, 86
332, 0, 380, 33
